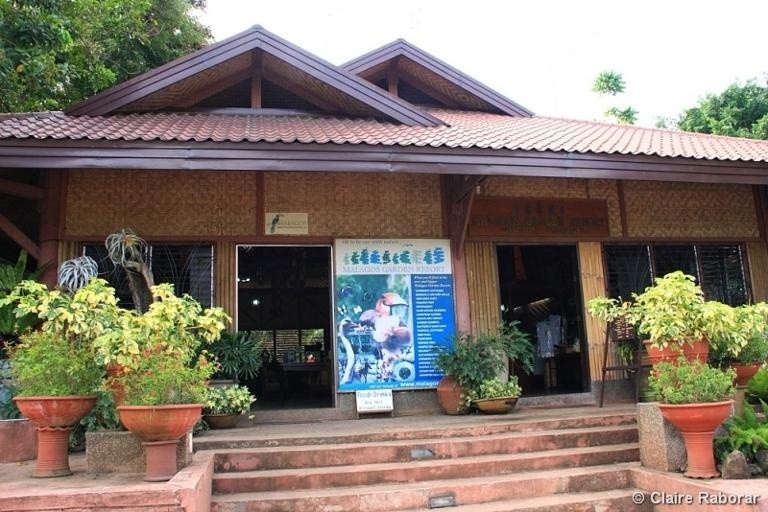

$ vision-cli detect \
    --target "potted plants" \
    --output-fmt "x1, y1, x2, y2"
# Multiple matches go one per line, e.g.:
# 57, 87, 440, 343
432, 321, 534, 414
4, 323, 100, 479
458, 376, 521, 414
621, 271, 709, 378
647, 350, 738, 480
202, 385, 257, 428
707, 300, 768, 385
0, 280, 231, 405
113, 345, 220, 482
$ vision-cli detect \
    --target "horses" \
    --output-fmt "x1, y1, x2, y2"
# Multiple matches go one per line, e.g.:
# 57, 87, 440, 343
359, 362, 372, 383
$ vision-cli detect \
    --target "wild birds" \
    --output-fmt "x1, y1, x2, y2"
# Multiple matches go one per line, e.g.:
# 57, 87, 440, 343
337, 316, 359, 384
